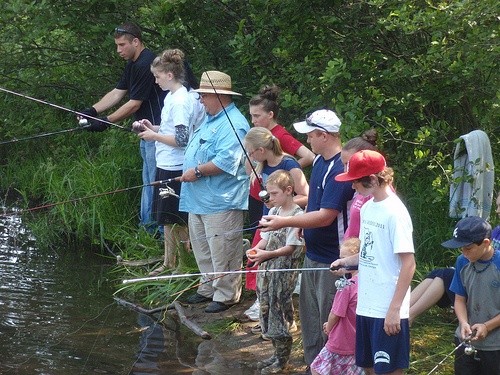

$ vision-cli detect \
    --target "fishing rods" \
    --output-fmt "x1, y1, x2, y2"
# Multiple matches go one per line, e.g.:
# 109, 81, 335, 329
0, 178, 180, 217
121, 265, 358, 283
180, 225, 264, 246
0, 89, 146, 133
0, 124, 92, 145
167, 252, 260, 298
172, 1, 269, 201
429, 332, 476, 375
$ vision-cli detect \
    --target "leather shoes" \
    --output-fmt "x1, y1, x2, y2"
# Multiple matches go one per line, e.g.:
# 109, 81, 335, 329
205, 301, 234, 312
185, 293, 206, 303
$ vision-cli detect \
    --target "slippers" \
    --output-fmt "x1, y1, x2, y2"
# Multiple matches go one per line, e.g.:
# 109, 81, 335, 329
149, 265, 171, 276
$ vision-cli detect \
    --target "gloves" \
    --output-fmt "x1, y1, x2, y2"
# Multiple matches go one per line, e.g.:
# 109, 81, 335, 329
88, 115, 111, 132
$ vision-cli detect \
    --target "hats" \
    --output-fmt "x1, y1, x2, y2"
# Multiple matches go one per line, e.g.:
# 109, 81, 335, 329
293, 109, 341, 133
441, 216, 492, 247
335, 149, 386, 181
192, 71, 242, 96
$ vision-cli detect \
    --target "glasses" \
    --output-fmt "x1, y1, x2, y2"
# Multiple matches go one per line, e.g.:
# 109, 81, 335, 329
114, 27, 139, 38
305, 113, 328, 133
247, 146, 263, 157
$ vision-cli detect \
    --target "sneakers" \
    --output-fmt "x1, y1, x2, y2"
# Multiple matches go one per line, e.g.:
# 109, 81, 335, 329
260, 321, 298, 341
251, 324, 261, 334
244, 299, 260, 322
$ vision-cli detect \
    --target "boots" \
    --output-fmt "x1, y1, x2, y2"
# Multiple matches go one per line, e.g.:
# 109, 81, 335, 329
257, 338, 294, 375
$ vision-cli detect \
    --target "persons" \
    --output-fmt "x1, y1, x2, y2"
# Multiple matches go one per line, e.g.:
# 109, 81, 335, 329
76, 22, 167, 241
247, 170, 306, 375
334, 149, 415, 375
244, 126, 310, 340
310, 238, 366, 375
138, 48, 207, 279
257, 109, 355, 375
244, 83, 316, 321
178, 70, 257, 315
340, 128, 396, 240
441, 214, 500, 375
489, 192, 500, 252
408, 267, 455, 326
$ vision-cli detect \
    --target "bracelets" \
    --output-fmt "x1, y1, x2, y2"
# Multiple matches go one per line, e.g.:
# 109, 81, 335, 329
483, 323, 490, 333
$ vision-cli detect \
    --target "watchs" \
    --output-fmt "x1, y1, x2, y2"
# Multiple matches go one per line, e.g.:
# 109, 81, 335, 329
194, 166, 204, 180
